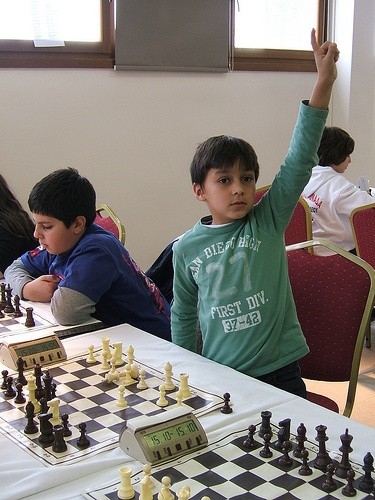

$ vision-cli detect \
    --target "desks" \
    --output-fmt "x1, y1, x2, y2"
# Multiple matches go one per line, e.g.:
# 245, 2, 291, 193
1, 300, 374, 499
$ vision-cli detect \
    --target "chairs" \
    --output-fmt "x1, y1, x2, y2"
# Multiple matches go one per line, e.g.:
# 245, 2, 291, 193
253, 183, 315, 255
285, 236, 375, 419
94, 204, 126, 247
349, 203, 375, 348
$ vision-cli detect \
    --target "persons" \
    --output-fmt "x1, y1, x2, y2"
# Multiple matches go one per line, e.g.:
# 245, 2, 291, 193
0, 174, 40, 279
172, 27, 340, 399
300, 127, 375, 256
3, 166, 171, 343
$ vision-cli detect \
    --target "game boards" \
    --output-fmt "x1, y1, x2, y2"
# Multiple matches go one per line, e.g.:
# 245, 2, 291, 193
82, 420, 375, 500
0, 345, 225, 468
0, 290, 59, 340
